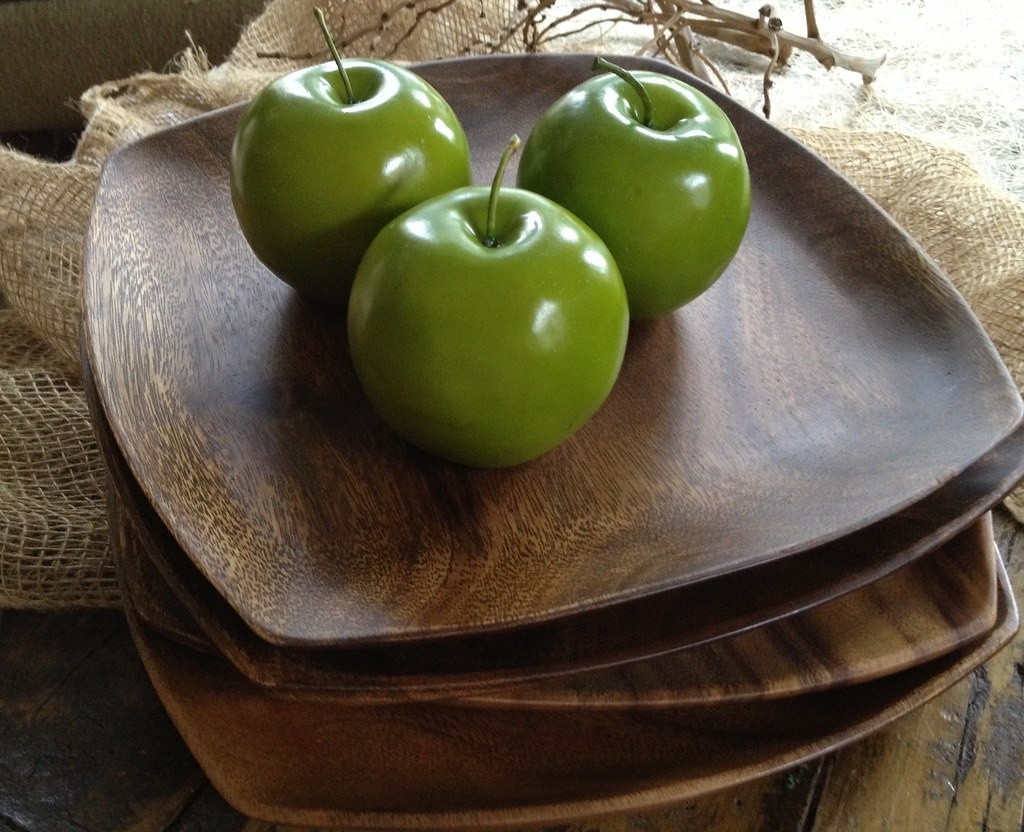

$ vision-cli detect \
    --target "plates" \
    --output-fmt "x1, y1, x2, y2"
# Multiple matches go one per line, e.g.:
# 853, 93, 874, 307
86, 52, 1024, 832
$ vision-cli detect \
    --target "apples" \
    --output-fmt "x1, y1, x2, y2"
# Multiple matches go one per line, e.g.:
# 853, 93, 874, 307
229, 4, 755, 474
